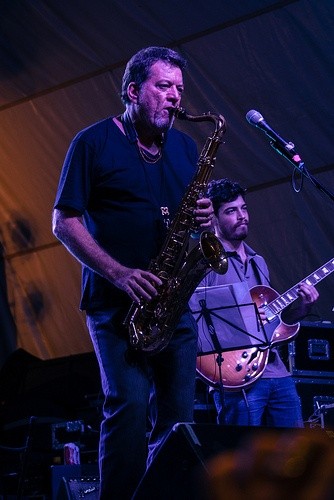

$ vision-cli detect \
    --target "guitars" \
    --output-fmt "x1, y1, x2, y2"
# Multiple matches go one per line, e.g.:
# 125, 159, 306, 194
196, 257, 334, 392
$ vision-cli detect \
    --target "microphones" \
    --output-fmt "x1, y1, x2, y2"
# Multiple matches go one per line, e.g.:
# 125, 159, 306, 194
246, 109, 294, 154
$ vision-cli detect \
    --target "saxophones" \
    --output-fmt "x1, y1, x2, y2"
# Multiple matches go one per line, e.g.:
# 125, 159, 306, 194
123, 104, 228, 353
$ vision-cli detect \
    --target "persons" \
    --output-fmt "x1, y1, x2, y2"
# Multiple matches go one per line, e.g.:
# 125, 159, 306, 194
188, 178, 321, 427
52, 46, 214, 500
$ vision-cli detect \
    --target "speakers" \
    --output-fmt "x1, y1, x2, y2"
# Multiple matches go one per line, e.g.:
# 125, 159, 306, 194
132, 423, 334, 500
